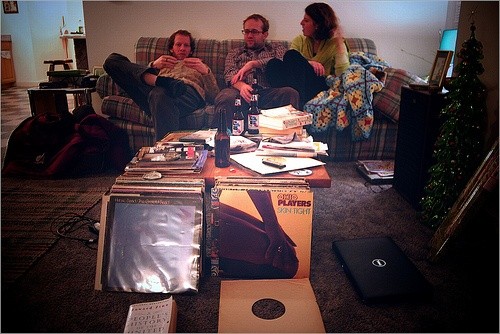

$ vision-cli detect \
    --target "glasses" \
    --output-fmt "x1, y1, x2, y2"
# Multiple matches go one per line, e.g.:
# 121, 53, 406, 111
241, 29, 263, 36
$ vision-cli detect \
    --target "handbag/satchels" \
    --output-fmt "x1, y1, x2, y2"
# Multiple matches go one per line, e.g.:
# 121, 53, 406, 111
3, 112, 73, 180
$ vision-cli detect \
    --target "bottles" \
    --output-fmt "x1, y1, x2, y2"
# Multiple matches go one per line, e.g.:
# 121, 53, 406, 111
214, 108, 230, 168
247, 78, 259, 134
79, 19, 83, 34
231, 95, 245, 135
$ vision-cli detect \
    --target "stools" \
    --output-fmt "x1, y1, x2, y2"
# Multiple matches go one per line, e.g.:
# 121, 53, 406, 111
42, 58, 73, 81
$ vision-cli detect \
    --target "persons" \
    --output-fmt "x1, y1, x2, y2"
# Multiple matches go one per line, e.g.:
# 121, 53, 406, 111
208, 2, 350, 128
103, 30, 220, 141
218, 190, 299, 279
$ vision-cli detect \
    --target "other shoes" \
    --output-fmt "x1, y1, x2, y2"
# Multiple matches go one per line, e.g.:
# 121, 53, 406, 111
168, 79, 188, 98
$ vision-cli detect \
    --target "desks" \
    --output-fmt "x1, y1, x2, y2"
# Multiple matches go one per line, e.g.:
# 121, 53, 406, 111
27, 86, 108, 119
125, 132, 331, 187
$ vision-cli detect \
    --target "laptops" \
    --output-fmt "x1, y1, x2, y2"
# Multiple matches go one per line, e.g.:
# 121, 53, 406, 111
331, 237, 435, 305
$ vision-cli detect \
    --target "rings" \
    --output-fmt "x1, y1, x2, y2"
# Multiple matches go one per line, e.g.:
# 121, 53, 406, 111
166, 58, 167, 61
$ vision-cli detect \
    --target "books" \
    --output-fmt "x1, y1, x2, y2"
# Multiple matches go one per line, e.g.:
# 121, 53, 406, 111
125, 105, 313, 174
123, 295, 178, 334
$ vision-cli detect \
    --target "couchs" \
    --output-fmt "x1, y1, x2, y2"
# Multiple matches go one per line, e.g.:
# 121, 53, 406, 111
96, 37, 428, 161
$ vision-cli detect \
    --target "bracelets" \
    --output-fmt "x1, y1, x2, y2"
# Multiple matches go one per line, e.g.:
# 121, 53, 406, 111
208, 67, 210, 73
308, 61, 311, 63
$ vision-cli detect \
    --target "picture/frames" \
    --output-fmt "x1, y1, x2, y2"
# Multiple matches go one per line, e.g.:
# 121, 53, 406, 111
2, 1, 19, 14
429, 49, 454, 86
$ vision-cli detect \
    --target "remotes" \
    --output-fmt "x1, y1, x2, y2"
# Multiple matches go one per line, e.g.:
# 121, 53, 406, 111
262, 158, 287, 168
